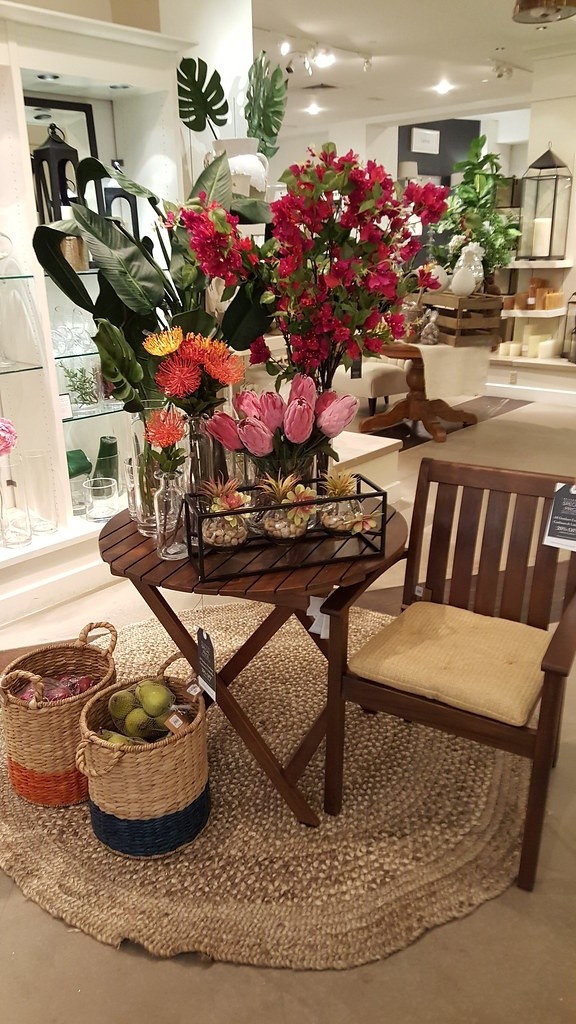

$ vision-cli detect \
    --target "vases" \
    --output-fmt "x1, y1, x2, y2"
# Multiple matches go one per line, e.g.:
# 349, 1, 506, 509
201, 496, 363, 555
451, 248, 484, 295
123, 460, 192, 561
247, 456, 313, 484
186, 416, 231, 484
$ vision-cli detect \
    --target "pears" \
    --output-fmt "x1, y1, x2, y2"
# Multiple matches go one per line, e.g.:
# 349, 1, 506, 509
96, 682, 181, 744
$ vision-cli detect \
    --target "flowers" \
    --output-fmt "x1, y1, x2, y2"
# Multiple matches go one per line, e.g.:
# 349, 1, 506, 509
320, 466, 384, 536
56, 145, 450, 470
251, 474, 317, 524
196, 471, 255, 526
422, 140, 523, 281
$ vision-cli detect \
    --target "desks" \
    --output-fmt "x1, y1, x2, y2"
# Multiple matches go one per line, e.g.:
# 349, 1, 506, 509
96, 487, 407, 826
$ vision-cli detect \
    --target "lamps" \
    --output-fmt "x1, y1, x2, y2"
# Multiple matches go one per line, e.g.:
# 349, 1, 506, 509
516, 140, 574, 261
33, 121, 90, 273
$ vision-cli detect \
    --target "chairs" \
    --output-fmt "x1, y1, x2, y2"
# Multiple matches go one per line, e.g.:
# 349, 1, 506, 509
324, 352, 412, 416
323, 457, 576, 892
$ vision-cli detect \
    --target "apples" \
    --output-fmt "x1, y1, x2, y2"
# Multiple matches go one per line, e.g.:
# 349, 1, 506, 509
17, 673, 95, 702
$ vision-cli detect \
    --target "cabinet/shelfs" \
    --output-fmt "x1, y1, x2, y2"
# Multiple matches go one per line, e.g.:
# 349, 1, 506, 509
1, 275, 144, 584
490, 258, 576, 370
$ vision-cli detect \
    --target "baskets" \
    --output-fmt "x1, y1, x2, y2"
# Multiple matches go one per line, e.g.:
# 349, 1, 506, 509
75, 650, 213, 859
0, 621, 117, 808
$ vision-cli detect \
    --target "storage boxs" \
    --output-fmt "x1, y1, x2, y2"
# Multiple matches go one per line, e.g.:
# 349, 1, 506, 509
405, 288, 502, 350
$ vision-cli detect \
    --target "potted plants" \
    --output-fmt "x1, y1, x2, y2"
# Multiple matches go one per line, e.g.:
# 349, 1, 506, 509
172, 51, 291, 202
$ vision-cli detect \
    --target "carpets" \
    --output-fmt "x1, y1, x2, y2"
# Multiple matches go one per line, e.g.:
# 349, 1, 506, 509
2, 601, 537, 968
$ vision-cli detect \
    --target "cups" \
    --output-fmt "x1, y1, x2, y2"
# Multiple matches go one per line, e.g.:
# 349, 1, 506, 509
69, 473, 94, 516
21, 448, 60, 537
82, 478, 119, 523
87, 450, 121, 500
0, 454, 32, 549
123, 457, 137, 521
